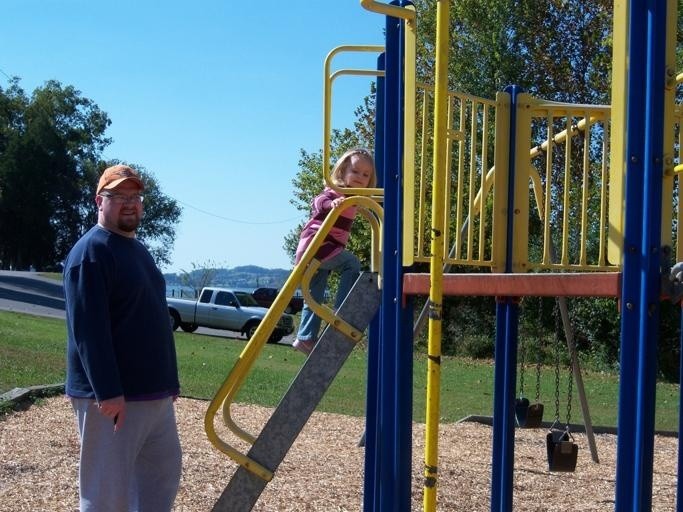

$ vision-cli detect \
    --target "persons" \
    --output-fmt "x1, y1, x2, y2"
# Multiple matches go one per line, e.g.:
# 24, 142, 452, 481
293, 147, 376, 359
64, 163, 183, 512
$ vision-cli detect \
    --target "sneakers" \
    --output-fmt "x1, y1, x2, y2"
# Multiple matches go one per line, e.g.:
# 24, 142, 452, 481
293, 339, 317, 355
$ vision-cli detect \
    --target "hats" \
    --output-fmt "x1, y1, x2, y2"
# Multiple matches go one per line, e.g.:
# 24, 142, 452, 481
97, 166, 145, 195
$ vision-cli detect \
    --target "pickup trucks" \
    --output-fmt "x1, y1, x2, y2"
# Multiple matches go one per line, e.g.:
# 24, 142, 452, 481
249, 287, 306, 316
165, 285, 295, 345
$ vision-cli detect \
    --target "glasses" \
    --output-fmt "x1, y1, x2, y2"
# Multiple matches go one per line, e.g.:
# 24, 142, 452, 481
102, 192, 146, 205
